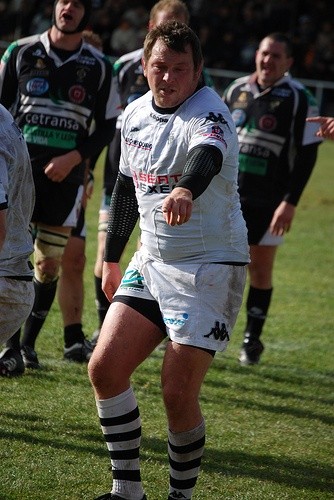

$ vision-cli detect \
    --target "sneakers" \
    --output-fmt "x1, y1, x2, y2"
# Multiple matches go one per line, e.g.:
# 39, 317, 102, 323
20, 346, 41, 370
0, 347, 26, 377
62, 339, 95, 364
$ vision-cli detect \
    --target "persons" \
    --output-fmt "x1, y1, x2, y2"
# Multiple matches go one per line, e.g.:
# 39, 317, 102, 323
306, 117, 334, 141
94, 18, 149, 56
0, 0, 117, 379
87, 21, 250, 500
221, 28, 321, 368
88, 0, 214, 347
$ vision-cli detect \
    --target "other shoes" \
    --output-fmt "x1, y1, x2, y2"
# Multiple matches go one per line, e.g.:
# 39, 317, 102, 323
238, 335, 264, 366
